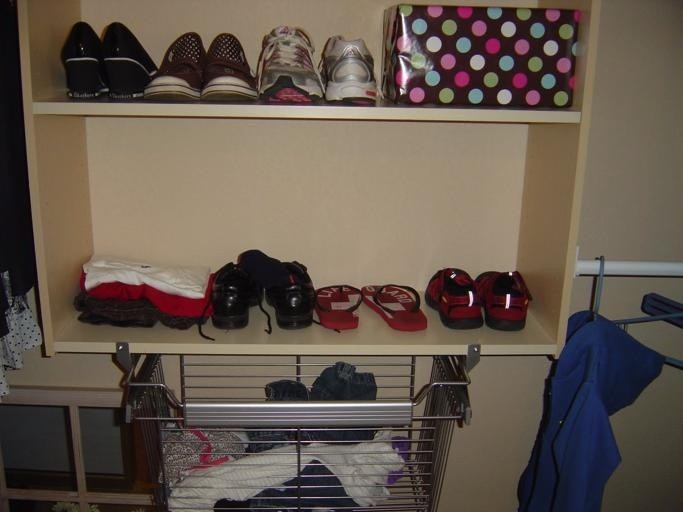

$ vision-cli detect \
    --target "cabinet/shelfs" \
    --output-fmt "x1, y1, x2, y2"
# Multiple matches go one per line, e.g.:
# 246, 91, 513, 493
0, 348, 154, 511
14, 2, 602, 359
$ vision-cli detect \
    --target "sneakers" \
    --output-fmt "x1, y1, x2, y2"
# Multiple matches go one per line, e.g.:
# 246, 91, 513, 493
200, 33, 256, 103
476, 271, 531, 331
317, 35, 378, 106
253, 25, 325, 104
424, 267, 483, 330
142, 32, 208, 103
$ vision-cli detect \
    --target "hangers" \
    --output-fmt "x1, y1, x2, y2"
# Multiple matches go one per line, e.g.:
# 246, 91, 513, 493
564, 255, 682, 385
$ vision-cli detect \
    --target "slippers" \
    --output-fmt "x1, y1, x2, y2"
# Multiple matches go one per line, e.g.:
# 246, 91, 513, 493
313, 285, 363, 328
359, 283, 428, 332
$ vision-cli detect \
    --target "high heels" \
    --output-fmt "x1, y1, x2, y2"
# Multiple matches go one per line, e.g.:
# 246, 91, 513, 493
99, 22, 157, 101
59, 22, 99, 100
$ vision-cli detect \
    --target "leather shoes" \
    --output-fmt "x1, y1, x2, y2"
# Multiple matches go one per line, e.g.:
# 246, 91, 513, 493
202, 262, 249, 329
265, 261, 315, 330
